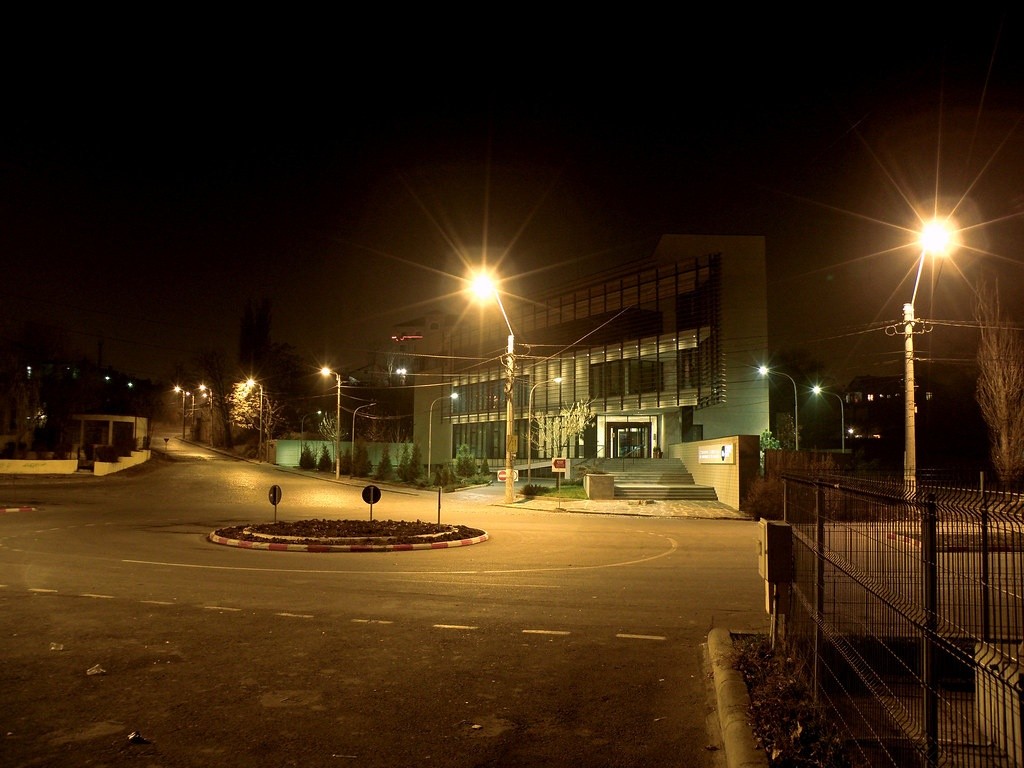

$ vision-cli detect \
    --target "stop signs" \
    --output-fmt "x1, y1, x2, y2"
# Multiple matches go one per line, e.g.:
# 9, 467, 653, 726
497, 469, 507, 482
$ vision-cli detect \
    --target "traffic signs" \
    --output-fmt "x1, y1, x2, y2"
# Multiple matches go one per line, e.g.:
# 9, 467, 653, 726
551, 458, 566, 472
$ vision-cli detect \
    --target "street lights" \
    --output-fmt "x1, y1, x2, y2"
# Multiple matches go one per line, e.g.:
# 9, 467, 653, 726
899, 216, 963, 521
351, 403, 377, 473
174, 386, 186, 437
320, 367, 341, 480
198, 384, 213, 449
812, 383, 845, 455
301, 410, 322, 457
528, 378, 565, 486
425, 392, 458, 480
464, 263, 515, 505
186, 391, 195, 429
247, 379, 262, 461
757, 366, 799, 453
